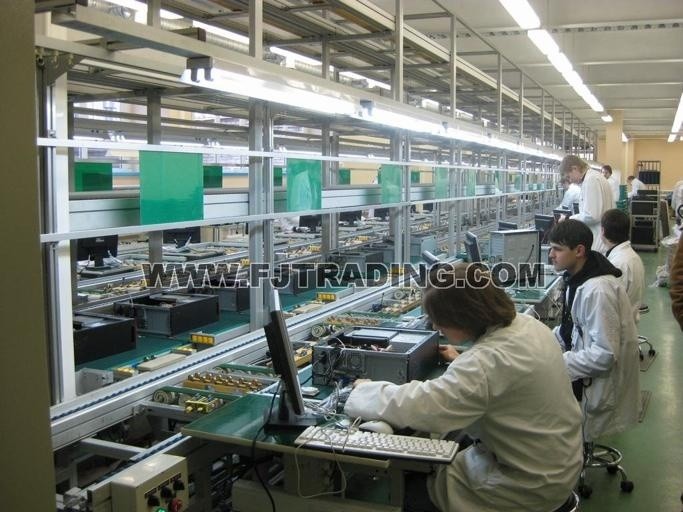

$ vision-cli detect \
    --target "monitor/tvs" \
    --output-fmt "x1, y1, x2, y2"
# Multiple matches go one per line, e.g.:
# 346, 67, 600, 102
339, 210, 361, 227
464, 231, 481, 264
497, 221, 517, 231
535, 214, 554, 244
78, 236, 118, 269
422, 250, 440, 271
299, 215, 322, 234
373, 208, 389, 222
163, 226, 200, 249
262, 289, 318, 430
553, 203, 579, 221
423, 203, 437, 212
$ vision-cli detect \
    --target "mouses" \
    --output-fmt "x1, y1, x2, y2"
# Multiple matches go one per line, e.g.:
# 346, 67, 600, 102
359, 421, 393, 434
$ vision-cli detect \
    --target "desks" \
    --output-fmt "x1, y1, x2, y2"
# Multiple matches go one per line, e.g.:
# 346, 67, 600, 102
180, 262, 567, 510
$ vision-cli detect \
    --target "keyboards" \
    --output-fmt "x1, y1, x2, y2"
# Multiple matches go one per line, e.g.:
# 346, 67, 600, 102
293, 425, 460, 464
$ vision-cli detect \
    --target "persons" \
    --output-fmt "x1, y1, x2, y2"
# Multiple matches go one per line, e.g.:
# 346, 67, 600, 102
668, 227, 683, 330
548, 155, 647, 442
343, 263, 583, 512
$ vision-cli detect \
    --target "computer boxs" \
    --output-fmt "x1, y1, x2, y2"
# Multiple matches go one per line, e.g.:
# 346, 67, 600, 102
312, 327, 439, 386
488, 228, 541, 286
72, 232, 436, 365
